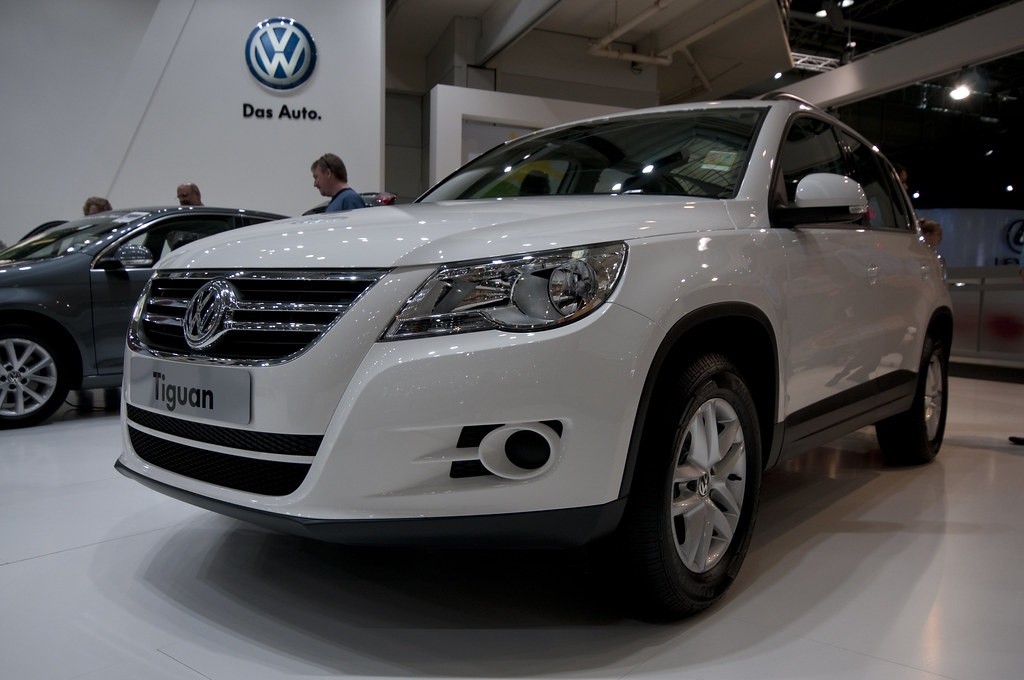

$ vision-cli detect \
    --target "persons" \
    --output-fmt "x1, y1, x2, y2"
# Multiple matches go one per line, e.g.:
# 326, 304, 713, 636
83, 196, 112, 220
311, 152, 367, 213
165, 182, 209, 252
889, 160, 943, 247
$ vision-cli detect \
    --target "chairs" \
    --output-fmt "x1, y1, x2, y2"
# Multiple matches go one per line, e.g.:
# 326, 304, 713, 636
621, 175, 688, 196
517, 170, 552, 196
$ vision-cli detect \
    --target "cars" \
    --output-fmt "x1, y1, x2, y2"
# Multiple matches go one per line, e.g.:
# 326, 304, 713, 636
0, 188, 397, 431
107, 90, 958, 620
